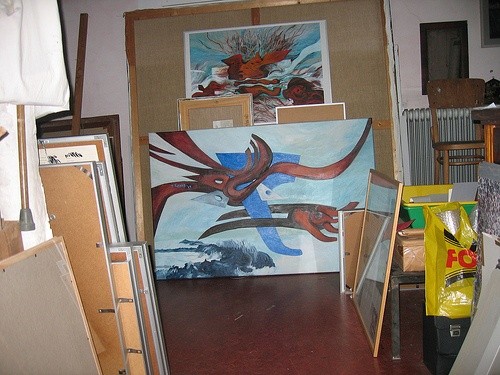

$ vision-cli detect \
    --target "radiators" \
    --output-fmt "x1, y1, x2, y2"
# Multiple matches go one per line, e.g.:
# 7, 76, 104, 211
402, 107, 478, 186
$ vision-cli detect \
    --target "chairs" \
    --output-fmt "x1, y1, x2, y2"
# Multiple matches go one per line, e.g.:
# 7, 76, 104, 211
426, 78, 485, 184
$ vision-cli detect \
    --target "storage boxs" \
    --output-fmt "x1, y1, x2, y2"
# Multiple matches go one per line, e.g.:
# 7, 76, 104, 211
398, 229, 425, 273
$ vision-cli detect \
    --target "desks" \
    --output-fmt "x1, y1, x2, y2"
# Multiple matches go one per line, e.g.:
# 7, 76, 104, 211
469, 110, 500, 166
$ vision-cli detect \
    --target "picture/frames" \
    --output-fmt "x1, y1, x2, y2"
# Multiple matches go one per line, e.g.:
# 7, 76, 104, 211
275, 102, 347, 126
1, 113, 172, 374
176, 93, 254, 132
479, 0, 500, 49
184, 17, 332, 127
352, 169, 405, 357
338, 210, 366, 294
420, 21, 470, 95
